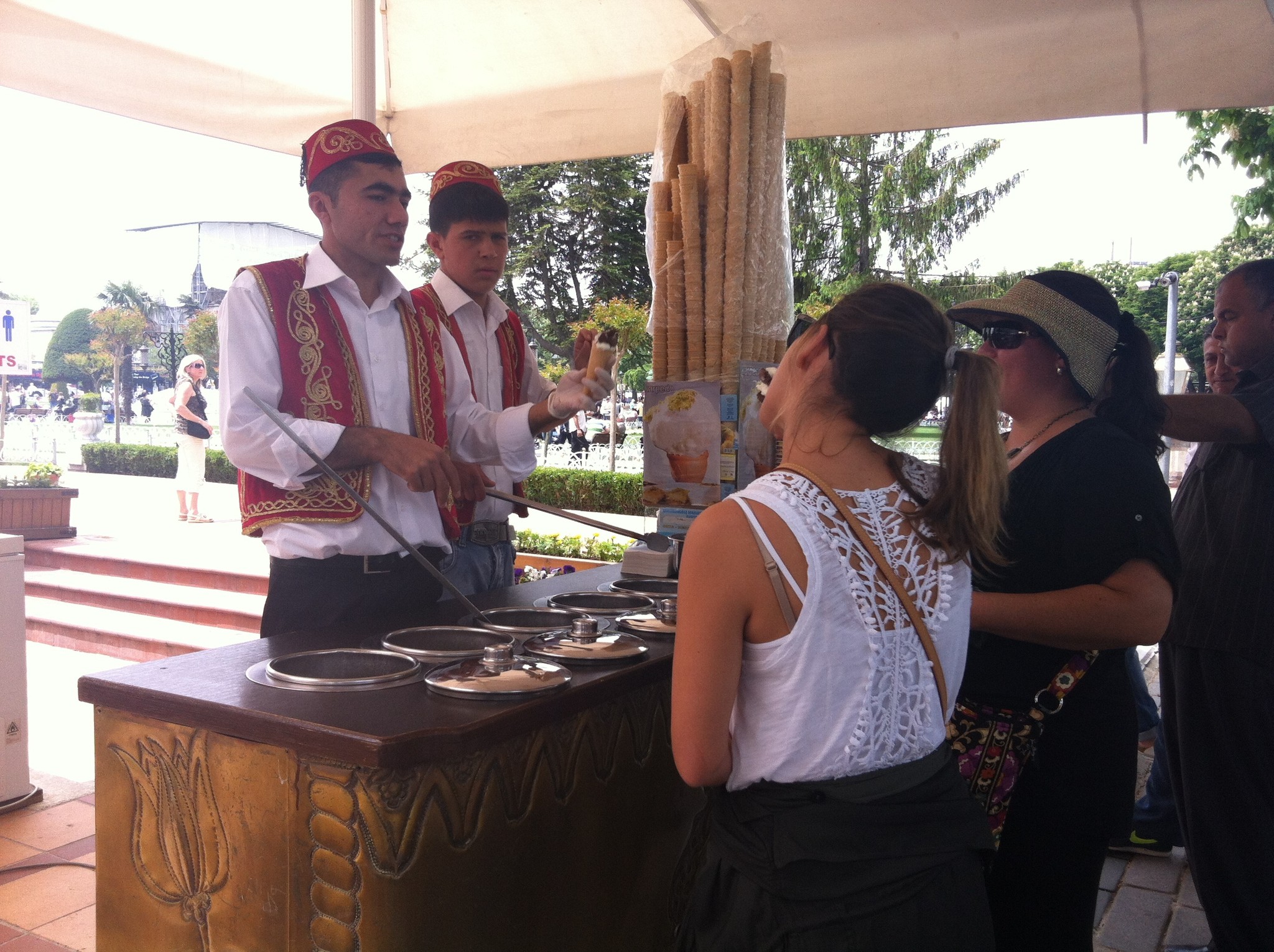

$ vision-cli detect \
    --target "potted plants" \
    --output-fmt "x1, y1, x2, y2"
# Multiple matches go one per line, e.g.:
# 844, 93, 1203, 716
72, 392, 106, 441
23, 462, 63, 488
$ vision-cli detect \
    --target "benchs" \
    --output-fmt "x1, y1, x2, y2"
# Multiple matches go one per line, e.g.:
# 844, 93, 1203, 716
13, 408, 49, 422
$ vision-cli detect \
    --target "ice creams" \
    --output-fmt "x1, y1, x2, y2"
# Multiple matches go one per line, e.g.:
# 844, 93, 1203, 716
583, 326, 618, 396
643, 389, 720, 484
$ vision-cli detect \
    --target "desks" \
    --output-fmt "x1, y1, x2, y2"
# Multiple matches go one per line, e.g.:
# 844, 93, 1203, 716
76, 562, 705, 951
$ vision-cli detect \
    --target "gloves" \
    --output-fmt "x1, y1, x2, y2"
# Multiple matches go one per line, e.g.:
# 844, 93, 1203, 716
548, 357, 617, 419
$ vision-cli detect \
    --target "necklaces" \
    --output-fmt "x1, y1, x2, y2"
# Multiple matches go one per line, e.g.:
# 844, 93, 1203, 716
1006, 404, 1089, 458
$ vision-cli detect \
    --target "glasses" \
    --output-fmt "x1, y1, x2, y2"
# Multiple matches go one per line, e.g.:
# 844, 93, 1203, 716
191, 363, 204, 368
976, 320, 1045, 351
788, 314, 835, 358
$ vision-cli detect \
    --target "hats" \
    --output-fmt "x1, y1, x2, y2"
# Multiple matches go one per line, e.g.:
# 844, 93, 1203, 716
302, 120, 399, 185
430, 160, 503, 200
946, 278, 1119, 400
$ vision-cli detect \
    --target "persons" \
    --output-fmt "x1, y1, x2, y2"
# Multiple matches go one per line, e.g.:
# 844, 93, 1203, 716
928, 267, 1177, 952
169, 354, 217, 523
407, 159, 604, 603
0, 375, 155, 425
669, 278, 1011, 952
216, 118, 620, 639
1108, 320, 1256, 859
3, 310, 14, 342
533, 383, 645, 466
1146, 256, 1274, 951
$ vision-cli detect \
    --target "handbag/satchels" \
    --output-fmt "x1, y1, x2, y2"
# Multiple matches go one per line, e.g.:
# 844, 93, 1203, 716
188, 420, 210, 439
943, 686, 1063, 854
148, 406, 154, 412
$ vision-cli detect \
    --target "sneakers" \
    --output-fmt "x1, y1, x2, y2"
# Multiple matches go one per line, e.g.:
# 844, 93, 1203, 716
1107, 816, 1173, 858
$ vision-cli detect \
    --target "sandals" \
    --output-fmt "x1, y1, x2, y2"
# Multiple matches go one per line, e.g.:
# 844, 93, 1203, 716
187, 513, 214, 523
178, 513, 189, 520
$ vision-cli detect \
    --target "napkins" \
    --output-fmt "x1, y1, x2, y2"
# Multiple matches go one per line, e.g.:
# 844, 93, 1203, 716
620, 544, 674, 578
